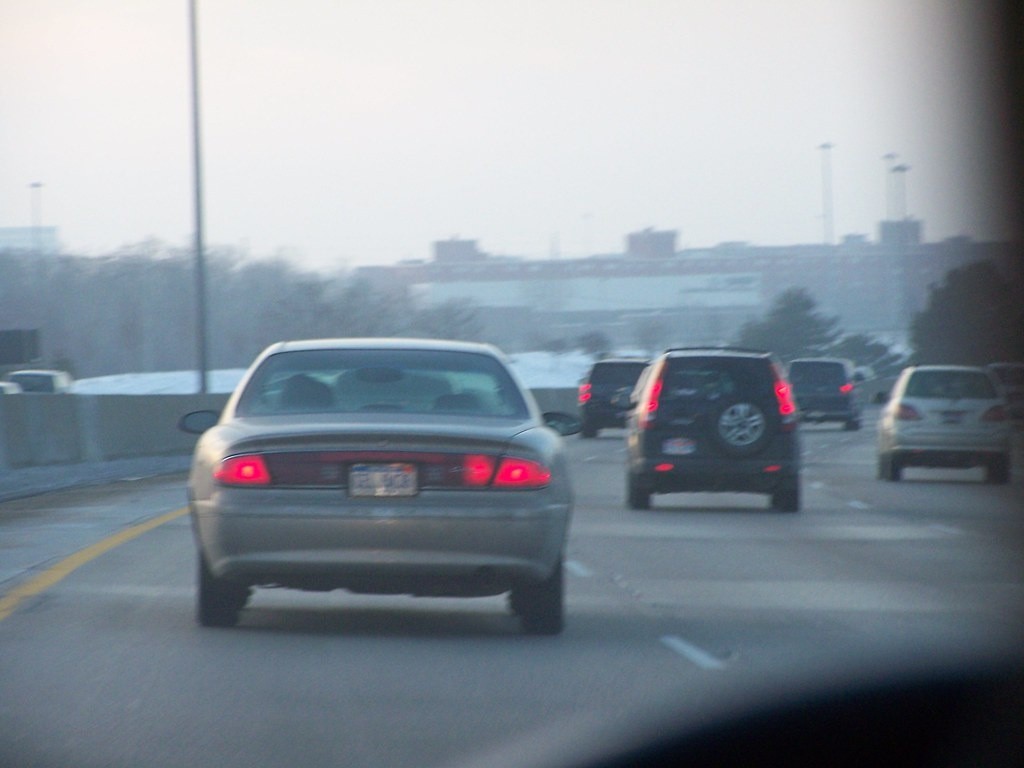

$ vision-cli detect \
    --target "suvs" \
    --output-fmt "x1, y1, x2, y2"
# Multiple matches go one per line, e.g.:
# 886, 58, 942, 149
625, 346, 804, 512
788, 358, 872, 430
579, 356, 651, 436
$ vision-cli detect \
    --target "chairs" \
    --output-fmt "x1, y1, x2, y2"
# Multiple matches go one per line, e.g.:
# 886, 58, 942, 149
280, 375, 333, 410
433, 394, 487, 412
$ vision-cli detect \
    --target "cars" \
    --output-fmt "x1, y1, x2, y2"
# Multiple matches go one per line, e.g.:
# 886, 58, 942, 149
874, 363, 1012, 482
179, 337, 576, 635
